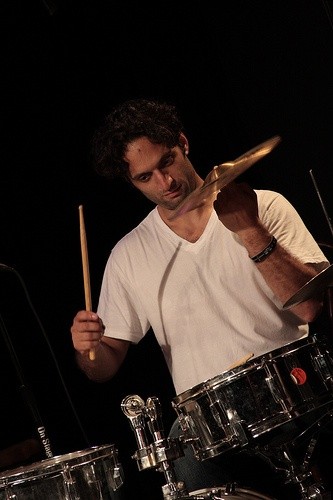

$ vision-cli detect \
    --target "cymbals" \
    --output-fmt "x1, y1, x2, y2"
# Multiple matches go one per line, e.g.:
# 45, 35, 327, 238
283, 264, 333, 308
167, 137, 279, 220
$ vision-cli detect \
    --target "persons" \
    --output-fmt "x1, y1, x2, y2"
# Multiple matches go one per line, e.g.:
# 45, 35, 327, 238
70, 100, 333, 454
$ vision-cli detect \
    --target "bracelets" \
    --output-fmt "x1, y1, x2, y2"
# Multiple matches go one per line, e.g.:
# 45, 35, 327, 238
247, 233, 276, 262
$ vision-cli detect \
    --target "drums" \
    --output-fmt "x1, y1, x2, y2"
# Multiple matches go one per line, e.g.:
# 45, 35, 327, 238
171, 333, 333, 462
0, 444, 123, 500
165, 482, 274, 500
